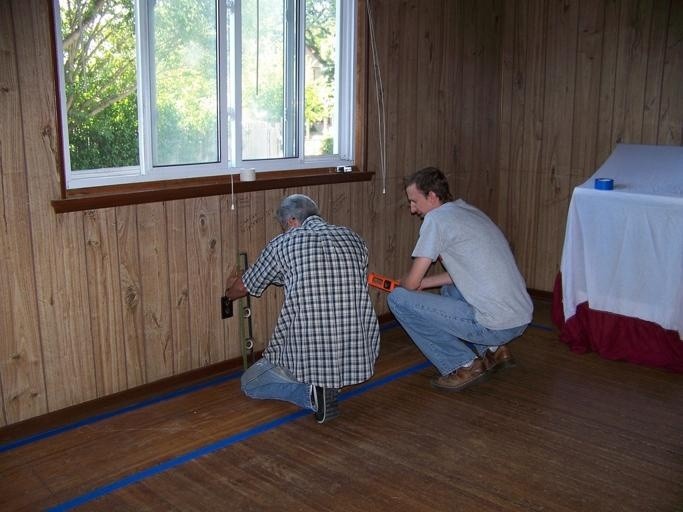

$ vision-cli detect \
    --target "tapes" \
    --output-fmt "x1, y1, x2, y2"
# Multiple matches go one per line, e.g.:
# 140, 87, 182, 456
595, 178, 614, 190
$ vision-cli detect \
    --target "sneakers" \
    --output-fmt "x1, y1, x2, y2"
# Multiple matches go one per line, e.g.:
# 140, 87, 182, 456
309, 382, 340, 424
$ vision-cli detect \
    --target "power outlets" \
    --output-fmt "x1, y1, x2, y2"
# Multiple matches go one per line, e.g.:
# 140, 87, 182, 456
222, 296, 234, 318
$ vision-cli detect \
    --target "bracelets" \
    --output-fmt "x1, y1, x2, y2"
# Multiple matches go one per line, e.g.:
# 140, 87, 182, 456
225, 288, 230, 298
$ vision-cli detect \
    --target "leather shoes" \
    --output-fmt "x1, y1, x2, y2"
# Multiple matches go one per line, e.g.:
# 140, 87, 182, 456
483, 345, 516, 376
430, 356, 488, 391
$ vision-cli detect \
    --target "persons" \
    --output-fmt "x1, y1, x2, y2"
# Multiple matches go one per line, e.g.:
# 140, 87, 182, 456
386, 165, 535, 394
223, 193, 383, 426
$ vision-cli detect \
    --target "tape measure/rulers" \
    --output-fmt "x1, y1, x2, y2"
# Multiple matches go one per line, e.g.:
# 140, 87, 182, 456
236, 252, 248, 369
